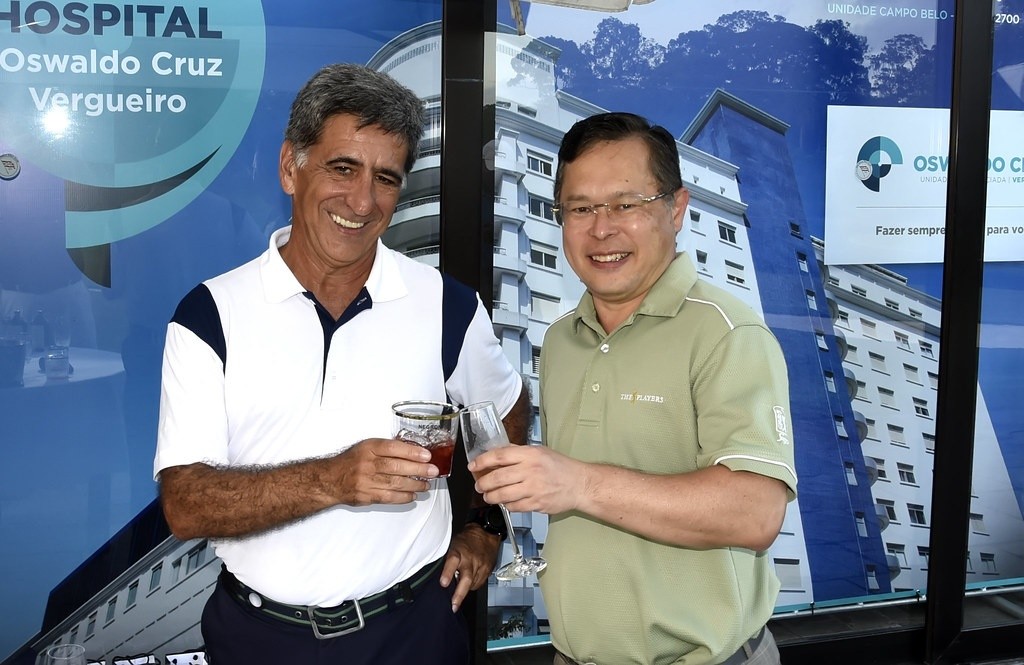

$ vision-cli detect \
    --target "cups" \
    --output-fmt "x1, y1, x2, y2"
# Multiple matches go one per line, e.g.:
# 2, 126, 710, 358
46, 644, 86, 665
392, 400, 460, 477
45, 346, 69, 379
0, 335, 26, 387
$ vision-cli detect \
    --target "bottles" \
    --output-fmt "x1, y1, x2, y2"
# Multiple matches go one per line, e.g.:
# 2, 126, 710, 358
8, 310, 27, 335
30, 310, 49, 349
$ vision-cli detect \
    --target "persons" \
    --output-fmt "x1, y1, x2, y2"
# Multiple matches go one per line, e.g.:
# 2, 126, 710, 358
469, 113, 797, 665
152, 63, 531, 664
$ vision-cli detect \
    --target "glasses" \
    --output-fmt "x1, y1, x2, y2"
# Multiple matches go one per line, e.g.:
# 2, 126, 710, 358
551, 187, 676, 220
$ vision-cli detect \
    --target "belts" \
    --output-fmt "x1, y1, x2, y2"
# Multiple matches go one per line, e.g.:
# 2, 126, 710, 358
219, 554, 446, 641
722, 625, 765, 665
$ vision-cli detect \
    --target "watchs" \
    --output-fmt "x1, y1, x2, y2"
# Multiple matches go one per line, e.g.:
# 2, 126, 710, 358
454, 507, 508, 542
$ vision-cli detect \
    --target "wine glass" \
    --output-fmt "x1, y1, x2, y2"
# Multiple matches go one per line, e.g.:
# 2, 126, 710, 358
459, 401, 547, 580
